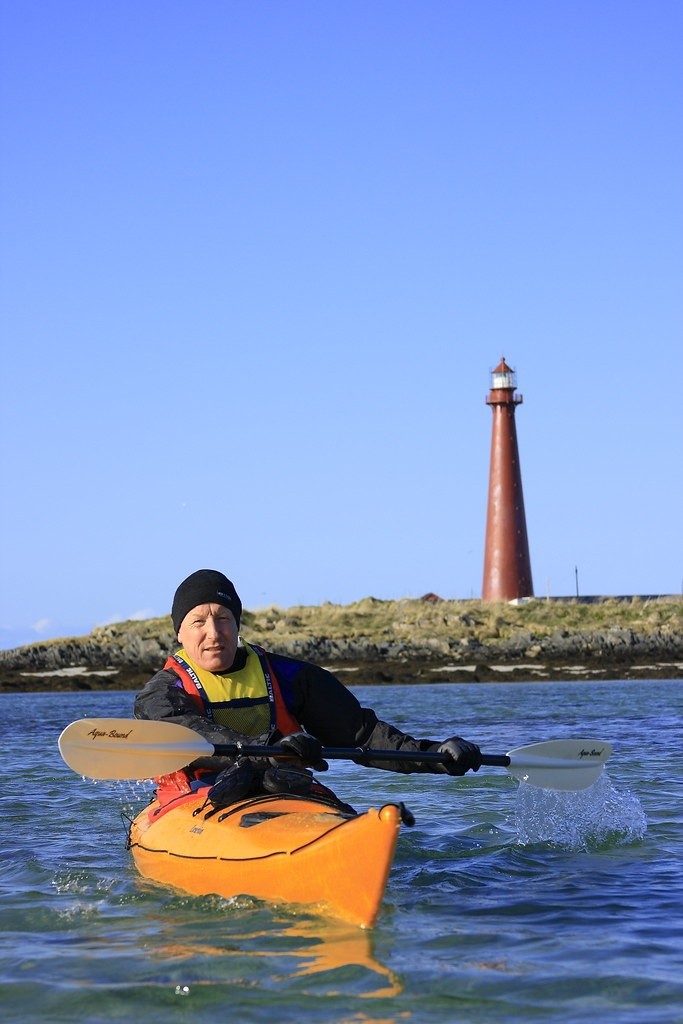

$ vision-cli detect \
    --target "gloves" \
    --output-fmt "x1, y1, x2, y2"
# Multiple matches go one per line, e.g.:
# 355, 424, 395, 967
433, 736, 483, 776
268, 732, 329, 771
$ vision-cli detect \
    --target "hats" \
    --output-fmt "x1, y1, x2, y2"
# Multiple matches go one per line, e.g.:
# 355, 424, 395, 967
170, 568, 242, 636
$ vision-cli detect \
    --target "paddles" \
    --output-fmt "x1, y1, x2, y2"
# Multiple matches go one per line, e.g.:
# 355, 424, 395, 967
56, 716, 614, 794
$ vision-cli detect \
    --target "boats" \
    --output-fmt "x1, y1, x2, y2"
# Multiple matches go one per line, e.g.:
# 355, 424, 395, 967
120, 774, 404, 932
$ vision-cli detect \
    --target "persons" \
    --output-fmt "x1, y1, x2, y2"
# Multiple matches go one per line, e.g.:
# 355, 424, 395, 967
133, 569, 484, 799
